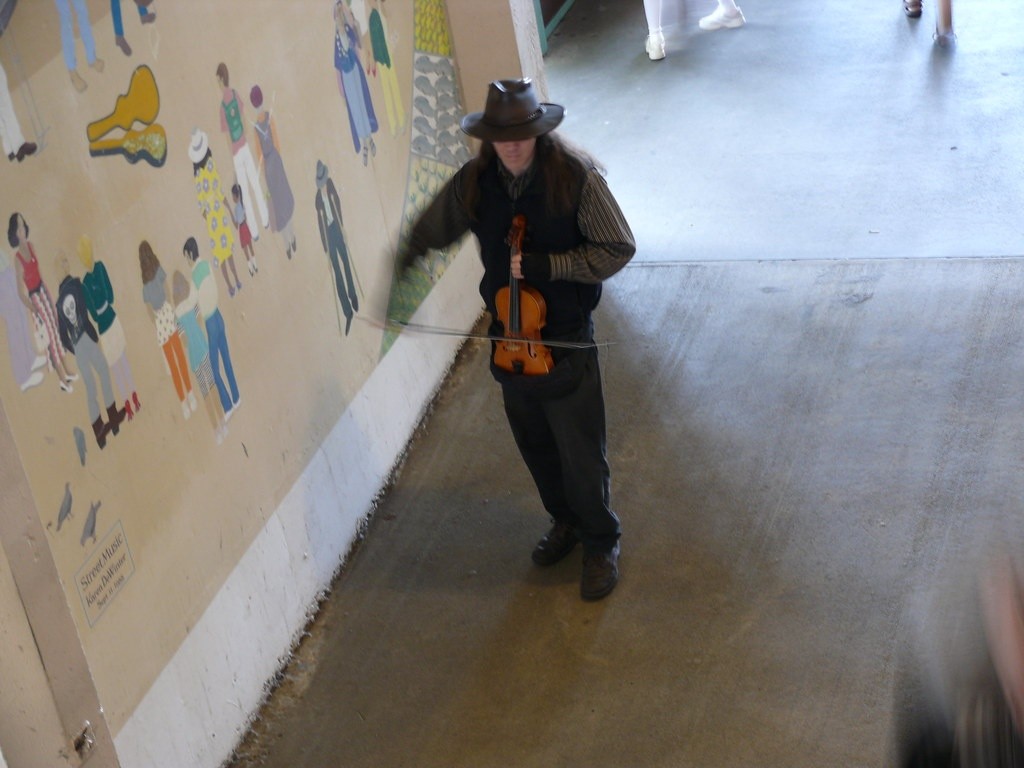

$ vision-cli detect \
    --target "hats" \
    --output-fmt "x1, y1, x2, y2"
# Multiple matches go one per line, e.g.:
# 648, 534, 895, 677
460, 79, 564, 142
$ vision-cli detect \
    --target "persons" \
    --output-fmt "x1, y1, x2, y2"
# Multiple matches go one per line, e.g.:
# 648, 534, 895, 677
372, 79, 637, 601
901, 0, 955, 47
643, 0, 745, 60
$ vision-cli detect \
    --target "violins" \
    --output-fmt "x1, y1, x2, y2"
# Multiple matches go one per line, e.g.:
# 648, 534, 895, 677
493, 216, 555, 375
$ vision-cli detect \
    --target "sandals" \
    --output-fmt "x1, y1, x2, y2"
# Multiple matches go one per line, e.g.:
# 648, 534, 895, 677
903, 1, 922, 18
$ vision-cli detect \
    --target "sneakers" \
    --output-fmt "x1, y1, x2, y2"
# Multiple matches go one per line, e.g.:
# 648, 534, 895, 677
646, 36, 666, 60
700, 6, 745, 30
582, 538, 620, 601
531, 518, 575, 565
